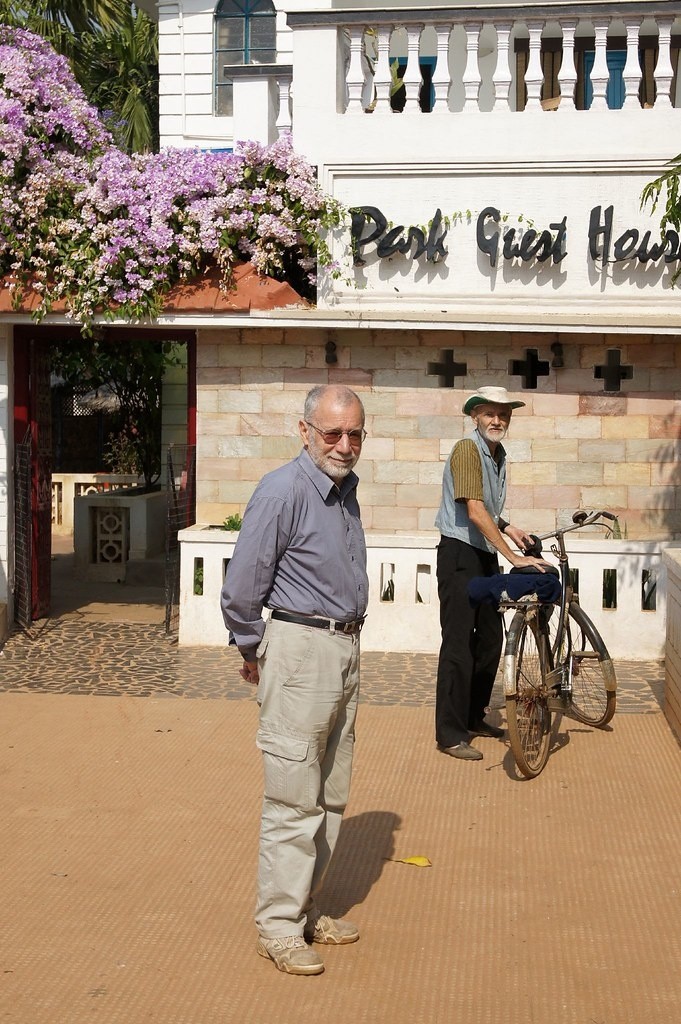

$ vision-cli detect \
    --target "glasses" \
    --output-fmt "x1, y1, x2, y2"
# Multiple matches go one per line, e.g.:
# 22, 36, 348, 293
305, 420, 368, 446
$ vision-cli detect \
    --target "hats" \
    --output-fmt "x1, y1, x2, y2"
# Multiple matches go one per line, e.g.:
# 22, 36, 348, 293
462, 386, 526, 416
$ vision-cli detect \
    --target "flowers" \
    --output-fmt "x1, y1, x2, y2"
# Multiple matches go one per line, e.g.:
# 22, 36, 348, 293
0, 21, 365, 340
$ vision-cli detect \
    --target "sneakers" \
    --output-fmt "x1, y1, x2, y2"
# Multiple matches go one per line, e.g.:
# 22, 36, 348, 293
256, 934, 324, 974
303, 913, 359, 944
469, 722, 504, 737
437, 741, 483, 761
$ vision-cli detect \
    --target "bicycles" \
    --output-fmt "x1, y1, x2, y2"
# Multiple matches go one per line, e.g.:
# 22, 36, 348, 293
493, 509, 621, 781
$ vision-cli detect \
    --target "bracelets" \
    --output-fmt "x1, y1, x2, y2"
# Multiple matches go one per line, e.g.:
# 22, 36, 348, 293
500, 523, 509, 532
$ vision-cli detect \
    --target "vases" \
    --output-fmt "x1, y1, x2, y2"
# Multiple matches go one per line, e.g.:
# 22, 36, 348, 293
0, 256, 313, 314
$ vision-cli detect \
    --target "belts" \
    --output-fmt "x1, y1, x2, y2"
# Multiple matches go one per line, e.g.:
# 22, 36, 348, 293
272, 610, 368, 634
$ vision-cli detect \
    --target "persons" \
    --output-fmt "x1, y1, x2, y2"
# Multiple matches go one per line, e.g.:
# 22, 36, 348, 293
434, 386, 553, 760
220, 384, 370, 974
